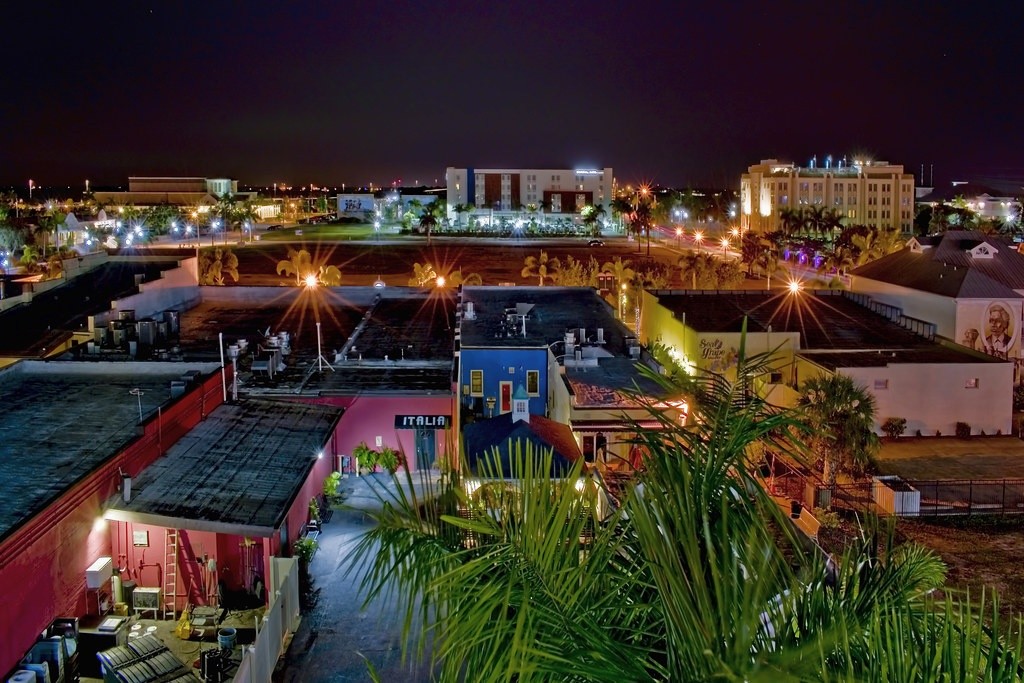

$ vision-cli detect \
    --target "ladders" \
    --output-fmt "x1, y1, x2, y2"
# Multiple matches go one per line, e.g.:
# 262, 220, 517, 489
163, 528, 177, 620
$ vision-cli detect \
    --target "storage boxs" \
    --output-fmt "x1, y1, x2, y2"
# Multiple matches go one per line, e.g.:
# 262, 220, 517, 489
86, 558, 114, 588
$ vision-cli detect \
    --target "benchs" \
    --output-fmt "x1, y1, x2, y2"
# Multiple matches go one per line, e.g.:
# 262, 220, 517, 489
189, 625, 223, 638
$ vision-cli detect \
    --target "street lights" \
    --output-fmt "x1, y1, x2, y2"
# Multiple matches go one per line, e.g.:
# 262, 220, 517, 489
695, 233, 701, 252
723, 239, 730, 262
677, 227, 682, 249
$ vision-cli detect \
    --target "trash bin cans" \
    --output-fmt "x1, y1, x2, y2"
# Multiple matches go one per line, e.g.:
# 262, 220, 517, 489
253, 234, 261, 241
295, 229, 302, 235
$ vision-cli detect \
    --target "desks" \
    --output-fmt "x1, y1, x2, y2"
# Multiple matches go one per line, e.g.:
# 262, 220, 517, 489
192, 606, 218, 627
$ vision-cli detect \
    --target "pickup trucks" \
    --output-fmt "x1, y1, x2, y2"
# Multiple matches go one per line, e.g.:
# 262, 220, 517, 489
310, 217, 323, 224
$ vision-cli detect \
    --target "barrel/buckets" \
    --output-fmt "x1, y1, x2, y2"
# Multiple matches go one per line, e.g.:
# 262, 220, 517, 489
218, 628, 237, 651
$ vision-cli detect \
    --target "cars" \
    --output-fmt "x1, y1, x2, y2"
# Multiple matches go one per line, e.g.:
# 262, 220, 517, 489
326, 214, 335, 220
267, 225, 282, 231
588, 240, 605, 247
